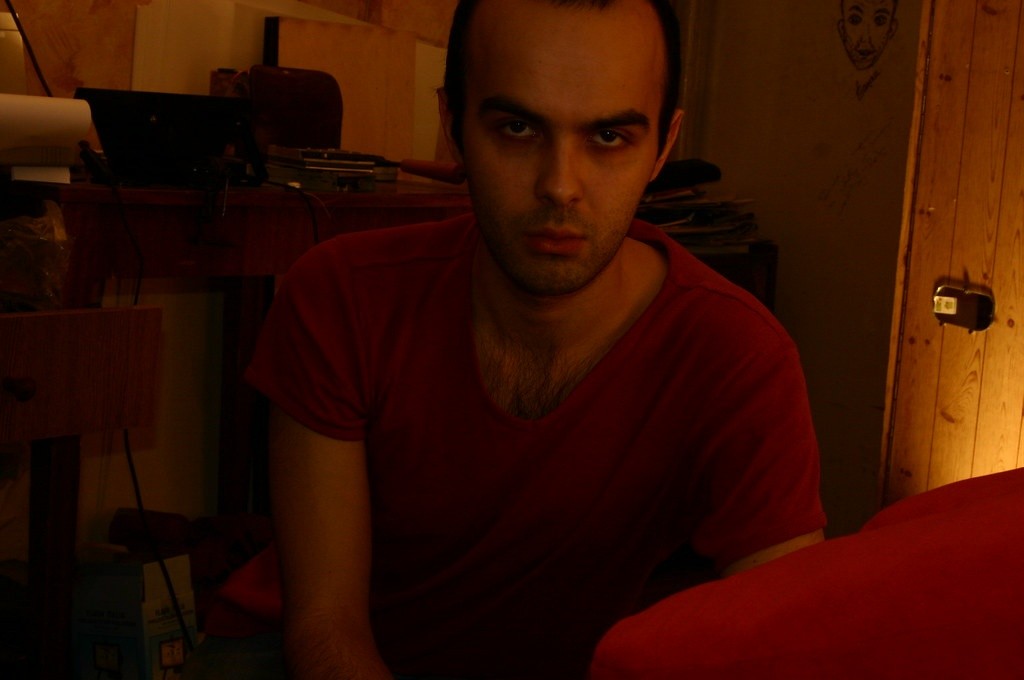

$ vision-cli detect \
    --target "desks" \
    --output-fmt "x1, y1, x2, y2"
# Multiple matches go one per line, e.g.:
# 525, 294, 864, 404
24, 164, 499, 680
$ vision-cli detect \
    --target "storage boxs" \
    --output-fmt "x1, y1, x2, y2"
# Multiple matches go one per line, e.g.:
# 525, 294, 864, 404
72, 555, 198, 680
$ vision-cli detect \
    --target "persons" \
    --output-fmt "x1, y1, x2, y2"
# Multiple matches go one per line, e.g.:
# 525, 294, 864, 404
242, 1, 828, 680
